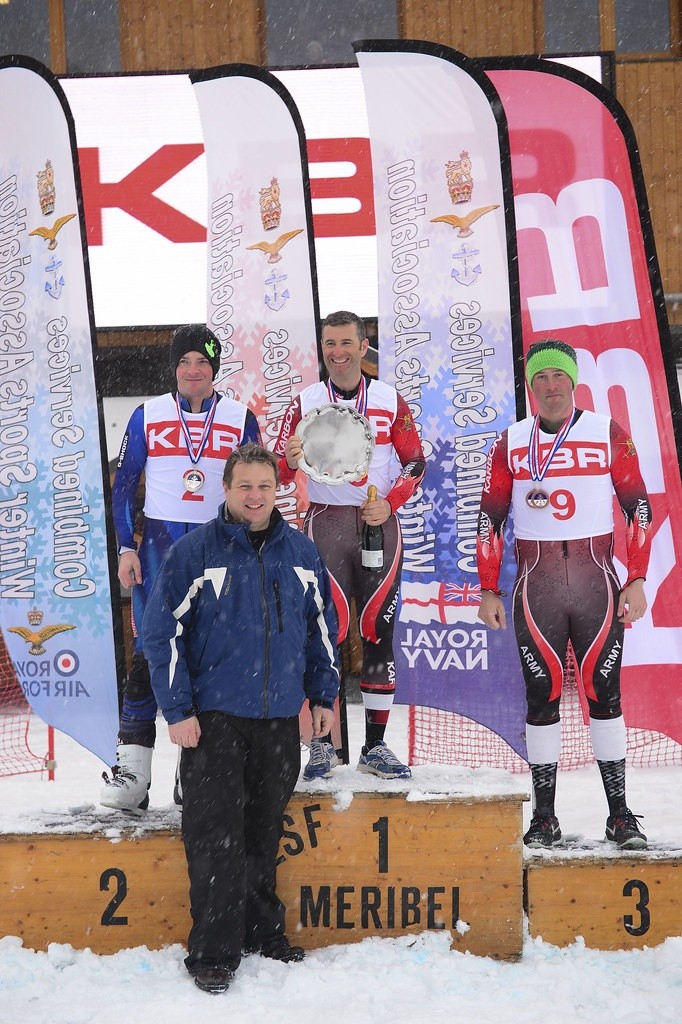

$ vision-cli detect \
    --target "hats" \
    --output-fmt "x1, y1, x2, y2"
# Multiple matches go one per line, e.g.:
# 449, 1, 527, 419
171, 325, 222, 381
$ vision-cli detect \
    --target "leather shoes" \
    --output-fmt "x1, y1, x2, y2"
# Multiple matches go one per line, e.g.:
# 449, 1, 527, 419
194, 970, 229, 992
260, 945, 305, 963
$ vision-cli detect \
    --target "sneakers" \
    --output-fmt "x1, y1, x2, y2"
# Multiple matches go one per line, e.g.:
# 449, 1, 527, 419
304, 737, 339, 779
603, 806, 648, 848
357, 740, 411, 779
524, 810, 563, 849
101, 743, 153, 816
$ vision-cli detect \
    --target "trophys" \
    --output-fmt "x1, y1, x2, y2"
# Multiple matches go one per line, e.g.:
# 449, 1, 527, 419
293, 403, 374, 485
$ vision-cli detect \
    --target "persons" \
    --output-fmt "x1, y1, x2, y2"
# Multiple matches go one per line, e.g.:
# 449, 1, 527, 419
140, 443, 340, 993
272, 311, 427, 781
476, 338, 652, 848
100, 325, 263, 816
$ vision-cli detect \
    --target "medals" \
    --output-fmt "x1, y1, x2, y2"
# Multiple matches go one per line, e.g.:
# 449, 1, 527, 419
349, 475, 368, 487
525, 488, 549, 509
183, 469, 205, 492
